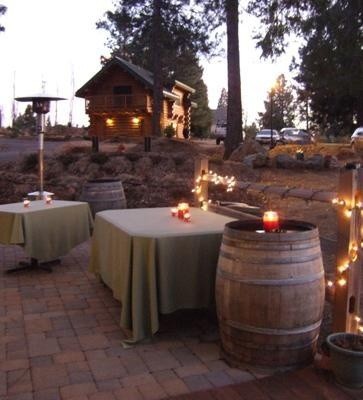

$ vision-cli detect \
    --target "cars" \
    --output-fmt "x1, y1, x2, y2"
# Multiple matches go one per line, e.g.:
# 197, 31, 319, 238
280, 127, 311, 144
255, 129, 282, 144
214, 122, 246, 145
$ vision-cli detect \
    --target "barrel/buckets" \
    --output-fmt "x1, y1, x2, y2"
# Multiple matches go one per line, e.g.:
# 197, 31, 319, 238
216, 217, 327, 374
78, 178, 128, 224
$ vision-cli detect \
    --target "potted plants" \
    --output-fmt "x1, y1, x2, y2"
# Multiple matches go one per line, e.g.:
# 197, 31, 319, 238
328, 330, 362, 390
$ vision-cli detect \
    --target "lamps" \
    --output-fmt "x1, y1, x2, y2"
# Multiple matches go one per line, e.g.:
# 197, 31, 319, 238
262, 212, 279, 233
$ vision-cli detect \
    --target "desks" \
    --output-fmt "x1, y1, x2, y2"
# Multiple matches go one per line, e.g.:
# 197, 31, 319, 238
0, 196, 242, 336
